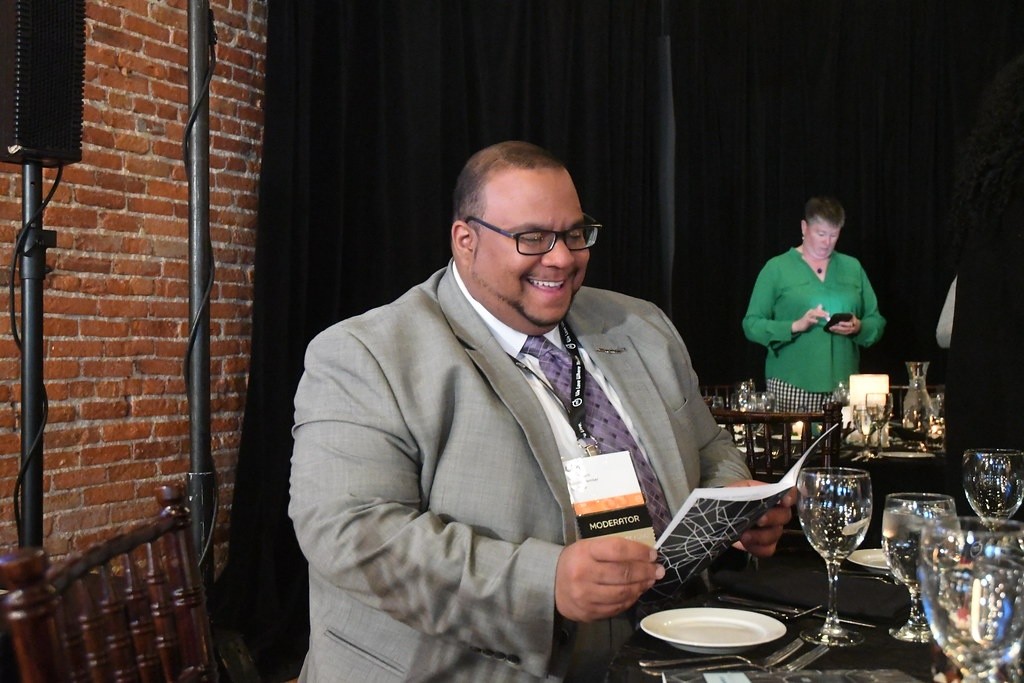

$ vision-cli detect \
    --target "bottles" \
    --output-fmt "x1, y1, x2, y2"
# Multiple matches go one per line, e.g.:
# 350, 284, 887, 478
903, 361, 930, 450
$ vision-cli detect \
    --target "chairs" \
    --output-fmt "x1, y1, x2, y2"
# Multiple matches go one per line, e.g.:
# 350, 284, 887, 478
889, 384, 946, 451
0, 480, 222, 683
700, 384, 740, 410
711, 402, 843, 535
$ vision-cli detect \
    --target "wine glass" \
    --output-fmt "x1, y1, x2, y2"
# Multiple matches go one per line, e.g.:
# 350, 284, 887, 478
960, 448, 1024, 531
866, 393, 892, 459
886, 493, 959, 642
740, 402, 765, 450
795, 468, 873, 646
853, 405, 878, 458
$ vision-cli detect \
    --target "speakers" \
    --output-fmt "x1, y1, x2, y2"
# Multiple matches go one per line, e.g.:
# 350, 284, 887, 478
0, 0, 87, 168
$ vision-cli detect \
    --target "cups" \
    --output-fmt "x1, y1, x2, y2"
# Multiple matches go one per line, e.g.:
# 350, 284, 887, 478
918, 517, 1024, 683
731, 381, 755, 412
832, 379, 850, 408
748, 392, 775, 413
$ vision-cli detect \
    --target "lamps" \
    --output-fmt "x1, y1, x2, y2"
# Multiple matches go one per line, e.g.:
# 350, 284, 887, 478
845, 374, 889, 448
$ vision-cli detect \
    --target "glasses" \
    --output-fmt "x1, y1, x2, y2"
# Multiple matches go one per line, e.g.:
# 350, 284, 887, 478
462, 212, 604, 256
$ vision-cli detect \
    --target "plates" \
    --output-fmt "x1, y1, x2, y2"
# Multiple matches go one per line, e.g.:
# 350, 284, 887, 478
846, 549, 890, 575
641, 607, 786, 655
880, 452, 935, 457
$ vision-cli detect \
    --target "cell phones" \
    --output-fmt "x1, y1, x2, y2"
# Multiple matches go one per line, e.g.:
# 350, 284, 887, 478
823, 313, 853, 331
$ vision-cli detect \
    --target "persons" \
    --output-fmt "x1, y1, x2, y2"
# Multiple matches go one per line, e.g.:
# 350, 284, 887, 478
742, 197, 889, 439
285, 140, 801, 683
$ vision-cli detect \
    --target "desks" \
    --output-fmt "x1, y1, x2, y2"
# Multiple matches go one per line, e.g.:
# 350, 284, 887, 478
605, 554, 956, 683
754, 420, 958, 550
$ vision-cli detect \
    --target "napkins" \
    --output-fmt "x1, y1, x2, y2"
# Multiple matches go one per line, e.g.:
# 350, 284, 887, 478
890, 425, 924, 441
722, 562, 910, 624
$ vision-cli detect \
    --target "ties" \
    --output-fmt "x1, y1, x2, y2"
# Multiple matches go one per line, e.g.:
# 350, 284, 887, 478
521, 335, 672, 540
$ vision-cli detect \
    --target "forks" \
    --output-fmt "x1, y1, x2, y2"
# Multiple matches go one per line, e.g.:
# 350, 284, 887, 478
637, 639, 803, 667
703, 604, 822, 619
641, 644, 829, 676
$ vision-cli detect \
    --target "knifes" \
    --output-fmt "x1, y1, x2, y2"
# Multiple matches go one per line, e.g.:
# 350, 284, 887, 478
719, 595, 876, 628
